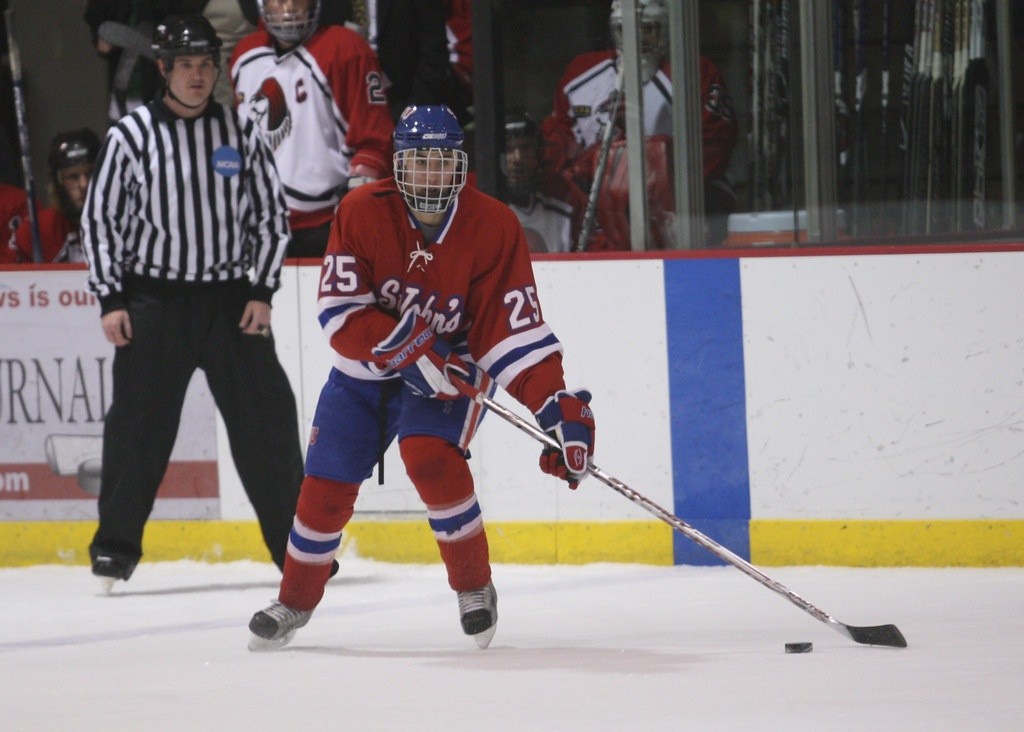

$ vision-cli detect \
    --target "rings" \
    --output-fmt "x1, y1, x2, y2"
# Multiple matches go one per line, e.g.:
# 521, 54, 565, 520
258, 324, 268, 333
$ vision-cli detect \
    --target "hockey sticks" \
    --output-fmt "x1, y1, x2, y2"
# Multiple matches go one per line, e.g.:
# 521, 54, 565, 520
446, 366, 909, 648
573, 52, 624, 253
4, 11, 47, 266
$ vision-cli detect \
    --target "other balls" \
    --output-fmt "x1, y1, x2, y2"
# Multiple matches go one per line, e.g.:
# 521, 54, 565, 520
784, 642, 812, 653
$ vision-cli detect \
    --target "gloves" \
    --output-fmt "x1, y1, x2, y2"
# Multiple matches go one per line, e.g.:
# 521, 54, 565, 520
338, 164, 385, 204
534, 390, 596, 489
371, 308, 472, 400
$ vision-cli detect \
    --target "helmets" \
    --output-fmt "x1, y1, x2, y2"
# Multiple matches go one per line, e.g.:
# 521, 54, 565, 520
611, 0, 669, 70
483, 111, 543, 200
392, 105, 468, 215
152, 14, 222, 55
258, 0, 321, 44
47, 129, 102, 176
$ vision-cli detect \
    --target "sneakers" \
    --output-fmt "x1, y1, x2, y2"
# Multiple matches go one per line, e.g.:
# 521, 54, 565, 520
249, 602, 311, 651
458, 578, 499, 647
91, 553, 126, 591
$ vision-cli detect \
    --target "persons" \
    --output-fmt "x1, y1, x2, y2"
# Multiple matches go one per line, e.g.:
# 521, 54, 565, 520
79, 13, 339, 598
469, 111, 594, 252
248, 101, 595, 649
0, 181, 44, 264
540, 0, 737, 252
16, 128, 101, 263
85, 0, 474, 256
